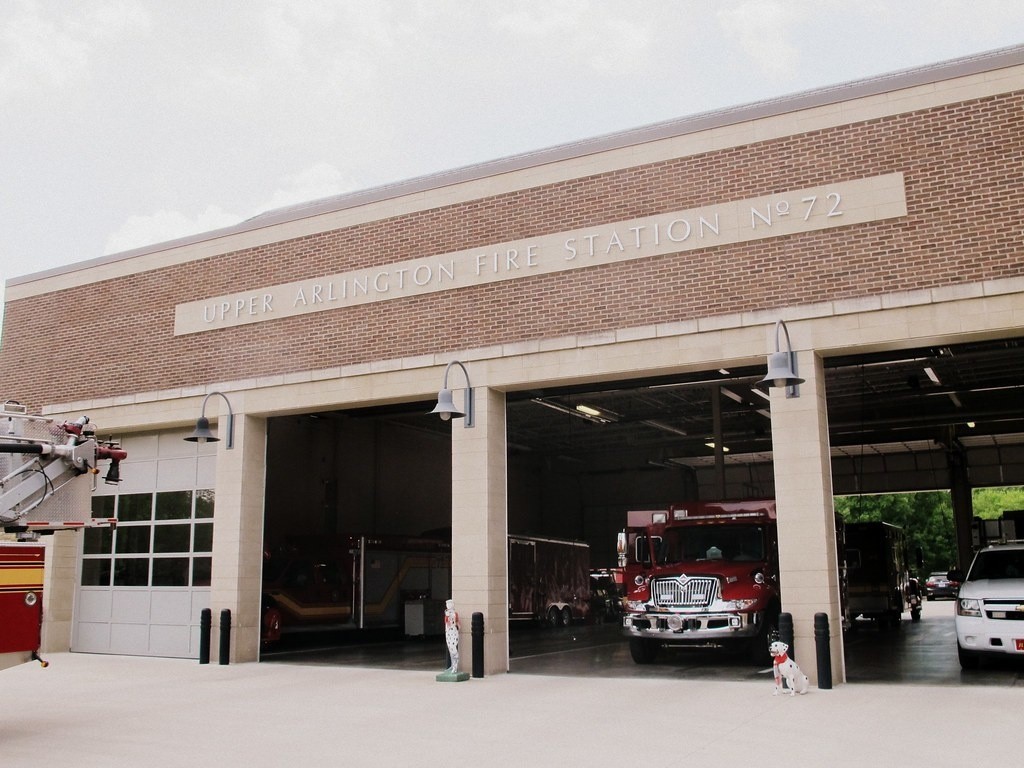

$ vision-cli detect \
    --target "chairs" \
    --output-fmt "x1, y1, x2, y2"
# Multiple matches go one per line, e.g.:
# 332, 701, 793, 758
735, 534, 761, 553
684, 535, 705, 560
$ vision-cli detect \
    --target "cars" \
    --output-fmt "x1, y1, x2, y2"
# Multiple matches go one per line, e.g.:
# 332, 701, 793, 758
946, 538, 1024, 673
915, 572, 950, 597
926, 575, 960, 601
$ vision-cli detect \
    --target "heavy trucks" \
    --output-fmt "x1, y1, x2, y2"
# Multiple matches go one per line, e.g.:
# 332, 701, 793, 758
507, 535, 622, 632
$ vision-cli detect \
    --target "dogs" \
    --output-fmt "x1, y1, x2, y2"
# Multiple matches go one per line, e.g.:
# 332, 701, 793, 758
768, 642, 809, 696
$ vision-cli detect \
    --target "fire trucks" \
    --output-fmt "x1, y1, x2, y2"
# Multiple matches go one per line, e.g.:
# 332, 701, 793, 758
1, 402, 129, 652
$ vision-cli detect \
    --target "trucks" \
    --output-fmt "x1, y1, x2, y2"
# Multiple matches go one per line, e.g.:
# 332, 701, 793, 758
844, 521, 926, 633
611, 502, 852, 665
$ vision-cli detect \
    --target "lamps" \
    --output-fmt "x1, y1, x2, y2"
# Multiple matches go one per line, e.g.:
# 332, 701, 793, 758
753, 318, 806, 399
183, 390, 235, 450
425, 359, 475, 429
